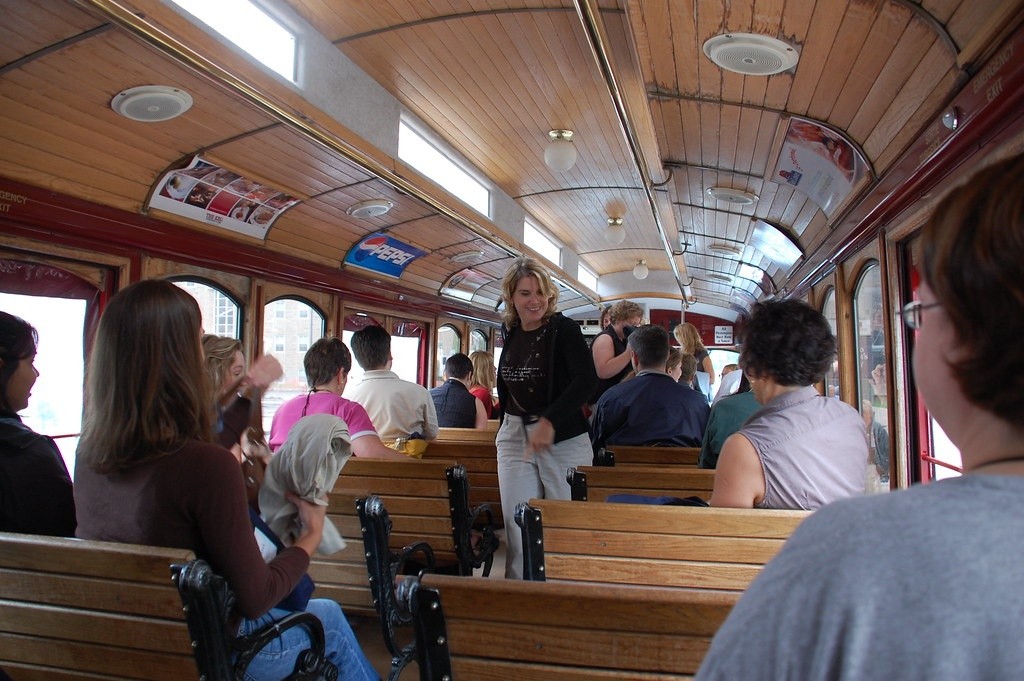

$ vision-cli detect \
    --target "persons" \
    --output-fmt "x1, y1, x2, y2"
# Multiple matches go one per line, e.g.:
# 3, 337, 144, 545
429, 350, 500, 429
74, 278, 439, 681
494, 257, 599, 581
0, 311, 77, 536
589, 298, 872, 510
185, 173, 255, 222
695, 155, 1024, 681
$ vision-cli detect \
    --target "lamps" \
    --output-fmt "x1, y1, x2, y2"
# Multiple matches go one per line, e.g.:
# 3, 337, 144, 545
605, 217, 625, 245
633, 258, 648, 279
544, 129, 577, 171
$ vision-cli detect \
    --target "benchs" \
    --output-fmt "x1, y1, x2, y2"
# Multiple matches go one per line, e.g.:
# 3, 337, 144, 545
1, 419, 816, 681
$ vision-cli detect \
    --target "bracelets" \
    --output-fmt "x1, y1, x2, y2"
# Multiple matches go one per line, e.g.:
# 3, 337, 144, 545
236, 390, 242, 398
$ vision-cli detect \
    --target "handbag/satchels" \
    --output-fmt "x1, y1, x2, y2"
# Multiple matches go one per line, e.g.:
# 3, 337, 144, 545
522, 406, 590, 446
245, 503, 315, 615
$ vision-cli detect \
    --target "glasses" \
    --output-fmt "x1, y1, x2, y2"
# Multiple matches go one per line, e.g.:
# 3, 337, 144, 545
894, 300, 941, 329
